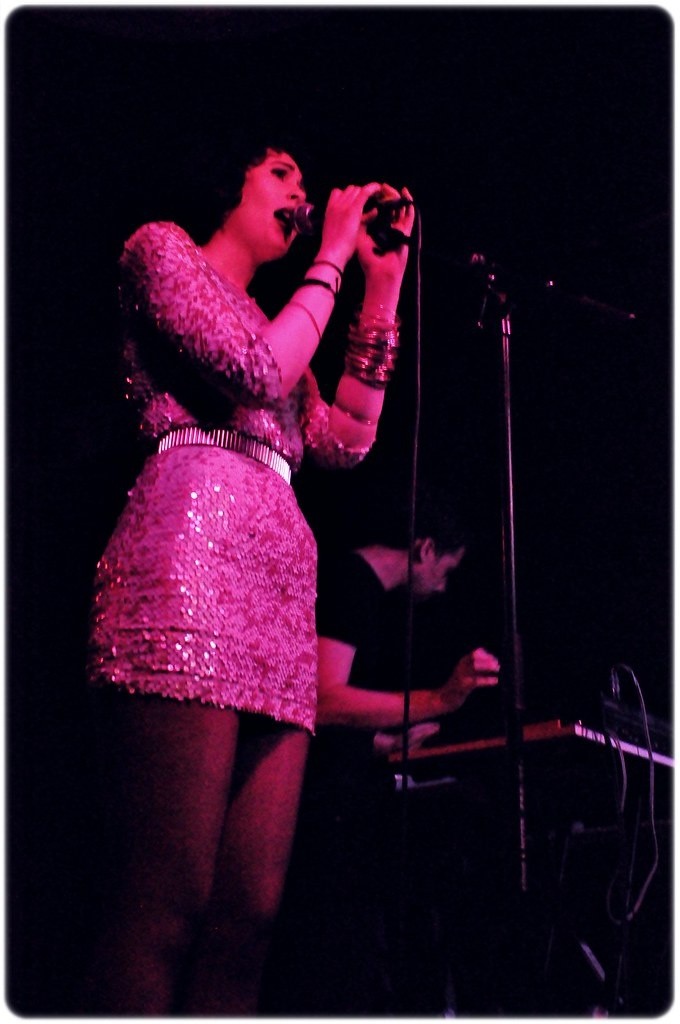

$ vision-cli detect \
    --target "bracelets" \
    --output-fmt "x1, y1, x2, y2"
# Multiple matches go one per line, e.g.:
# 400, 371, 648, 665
306, 255, 348, 282
342, 319, 401, 389
297, 275, 339, 294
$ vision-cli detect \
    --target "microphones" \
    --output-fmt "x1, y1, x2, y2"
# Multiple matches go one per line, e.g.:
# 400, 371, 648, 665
294, 196, 412, 237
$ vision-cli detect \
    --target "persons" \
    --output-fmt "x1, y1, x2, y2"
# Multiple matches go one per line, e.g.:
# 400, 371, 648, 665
83, 127, 415, 1016
230, 479, 505, 917
